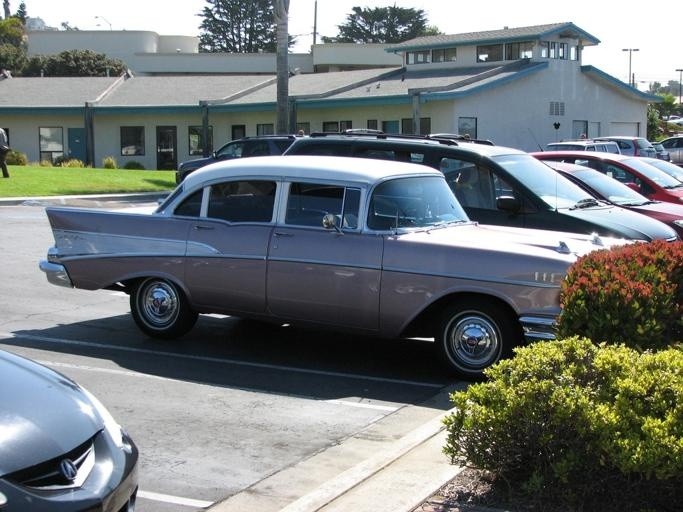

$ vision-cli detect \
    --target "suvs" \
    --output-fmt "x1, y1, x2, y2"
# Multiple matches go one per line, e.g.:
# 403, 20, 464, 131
175, 134, 304, 185
281, 128, 681, 252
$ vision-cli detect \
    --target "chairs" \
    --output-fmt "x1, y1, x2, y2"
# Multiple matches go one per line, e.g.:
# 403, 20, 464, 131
221, 193, 273, 222
298, 187, 374, 225
454, 166, 487, 209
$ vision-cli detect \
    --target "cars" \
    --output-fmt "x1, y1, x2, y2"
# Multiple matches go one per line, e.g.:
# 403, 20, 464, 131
494, 133, 681, 226
38, 155, 644, 382
659, 115, 682, 129
0, 347, 140, 509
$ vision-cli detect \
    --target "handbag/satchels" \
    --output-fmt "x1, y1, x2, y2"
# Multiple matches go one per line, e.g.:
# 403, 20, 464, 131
1, 145, 10, 161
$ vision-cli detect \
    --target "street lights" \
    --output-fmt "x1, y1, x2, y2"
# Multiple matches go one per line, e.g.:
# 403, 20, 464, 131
622, 48, 640, 89
675, 70, 683, 107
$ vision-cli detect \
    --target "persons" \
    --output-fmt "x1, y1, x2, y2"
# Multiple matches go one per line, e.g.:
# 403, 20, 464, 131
0, 126, 10, 179
